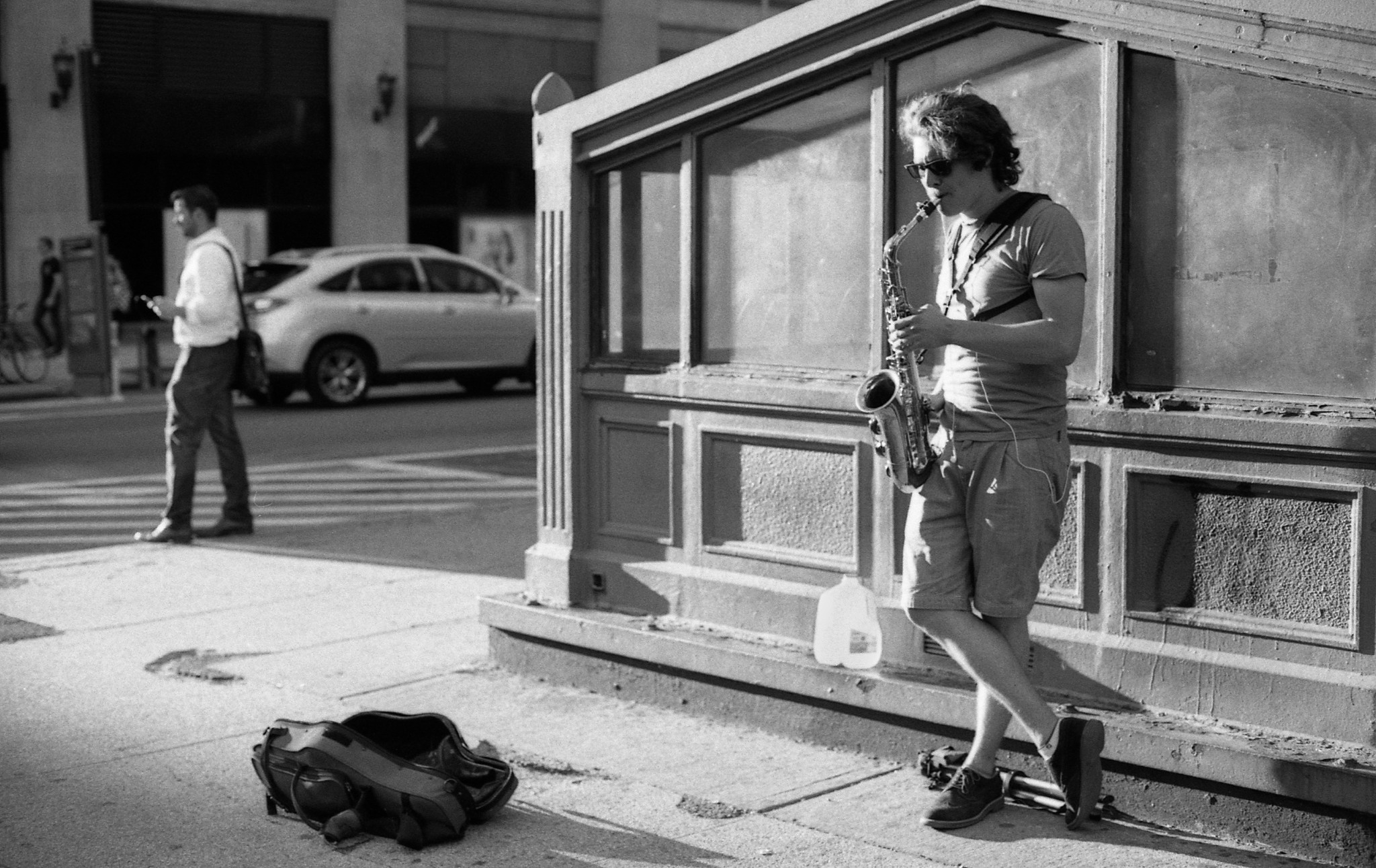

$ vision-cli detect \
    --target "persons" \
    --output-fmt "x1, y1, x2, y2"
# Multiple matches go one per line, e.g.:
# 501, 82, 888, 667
889, 79, 1105, 831
135, 188, 253, 541
33, 237, 64, 355
101, 236, 131, 351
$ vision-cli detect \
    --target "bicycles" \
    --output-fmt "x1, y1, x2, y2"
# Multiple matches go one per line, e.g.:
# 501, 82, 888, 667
0, 302, 53, 387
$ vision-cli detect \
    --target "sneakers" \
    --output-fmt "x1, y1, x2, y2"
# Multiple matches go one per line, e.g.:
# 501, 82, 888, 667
921, 767, 1003, 828
1037, 717, 1105, 829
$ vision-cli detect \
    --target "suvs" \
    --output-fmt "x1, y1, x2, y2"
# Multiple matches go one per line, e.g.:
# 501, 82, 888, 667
233, 241, 537, 408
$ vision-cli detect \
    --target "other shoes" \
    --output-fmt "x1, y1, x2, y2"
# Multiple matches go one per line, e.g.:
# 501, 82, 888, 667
197, 516, 253, 537
134, 518, 192, 542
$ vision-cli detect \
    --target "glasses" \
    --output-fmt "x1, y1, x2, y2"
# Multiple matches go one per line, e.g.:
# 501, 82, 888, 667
904, 158, 970, 178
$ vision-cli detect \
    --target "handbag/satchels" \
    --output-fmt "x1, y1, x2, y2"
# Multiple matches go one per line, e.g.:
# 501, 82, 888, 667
232, 331, 273, 401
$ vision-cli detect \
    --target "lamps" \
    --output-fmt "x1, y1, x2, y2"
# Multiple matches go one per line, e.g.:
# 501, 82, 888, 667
373, 73, 397, 122
50, 36, 74, 109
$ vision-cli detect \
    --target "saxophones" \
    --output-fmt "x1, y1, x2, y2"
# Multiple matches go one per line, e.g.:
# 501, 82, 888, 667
855, 197, 947, 493
